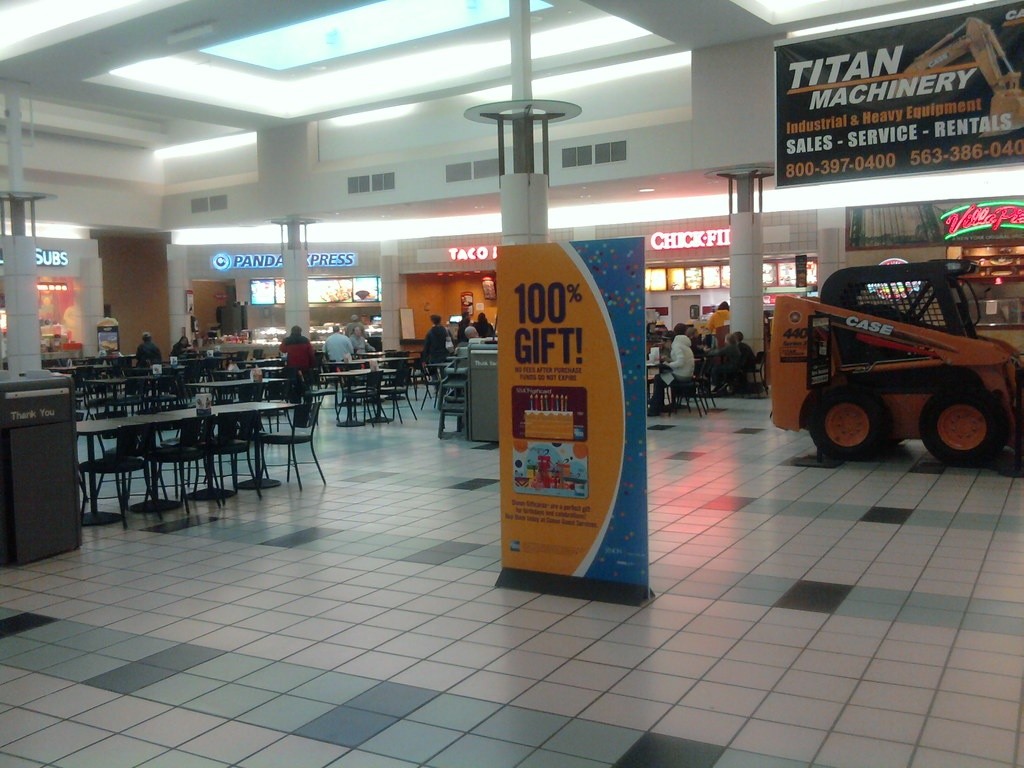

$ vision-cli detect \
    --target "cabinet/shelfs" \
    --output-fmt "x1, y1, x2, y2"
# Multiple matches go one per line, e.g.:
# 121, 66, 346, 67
958, 245, 1024, 282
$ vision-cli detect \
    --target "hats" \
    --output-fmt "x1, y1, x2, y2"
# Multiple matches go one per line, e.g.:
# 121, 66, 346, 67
659, 330, 674, 340
351, 314, 359, 322
291, 325, 302, 332
142, 331, 150, 338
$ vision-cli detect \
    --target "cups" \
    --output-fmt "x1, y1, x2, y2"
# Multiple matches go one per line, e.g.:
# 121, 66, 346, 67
649, 354, 655, 364
575, 484, 584, 496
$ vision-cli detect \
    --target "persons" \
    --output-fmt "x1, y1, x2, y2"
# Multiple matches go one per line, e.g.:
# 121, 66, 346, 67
972, 288, 1007, 323
322, 314, 376, 387
646, 301, 756, 413
421, 311, 494, 382
169, 337, 192, 358
279, 326, 315, 377
136, 332, 161, 367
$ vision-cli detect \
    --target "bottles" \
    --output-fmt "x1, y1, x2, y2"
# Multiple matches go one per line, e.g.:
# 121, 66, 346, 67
531, 468, 560, 489
817, 341, 826, 365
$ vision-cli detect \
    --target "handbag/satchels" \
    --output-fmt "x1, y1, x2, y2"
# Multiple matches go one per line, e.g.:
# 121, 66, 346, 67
445, 327, 455, 354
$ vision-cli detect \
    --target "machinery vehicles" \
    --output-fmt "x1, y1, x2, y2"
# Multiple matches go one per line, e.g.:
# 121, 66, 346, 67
770, 260, 1024, 466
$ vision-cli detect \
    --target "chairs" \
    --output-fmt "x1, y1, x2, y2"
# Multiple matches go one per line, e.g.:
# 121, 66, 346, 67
742, 352, 768, 398
666, 353, 716, 418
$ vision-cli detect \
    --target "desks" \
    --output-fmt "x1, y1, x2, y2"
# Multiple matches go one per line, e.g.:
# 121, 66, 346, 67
200, 351, 237, 355
170, 356, 227, 362
321, 371, 366, 427
646, 358, 701, 367
52, 347, 467, 532
183, 403, 236, 502
132, 365, 186, 370
112, 414, 182, 515
74, 410, 122, 527
236, 358, 284, 365
48, 365, 109, 370
99, 353, 136, 360
186, 378, 287, 402
85, 375, 166, 384
323, 357, 415, 365
352, 352, 386, 357
235, 401, 298, 489
351, 369, 396, 423
215, 367, 283, 376
